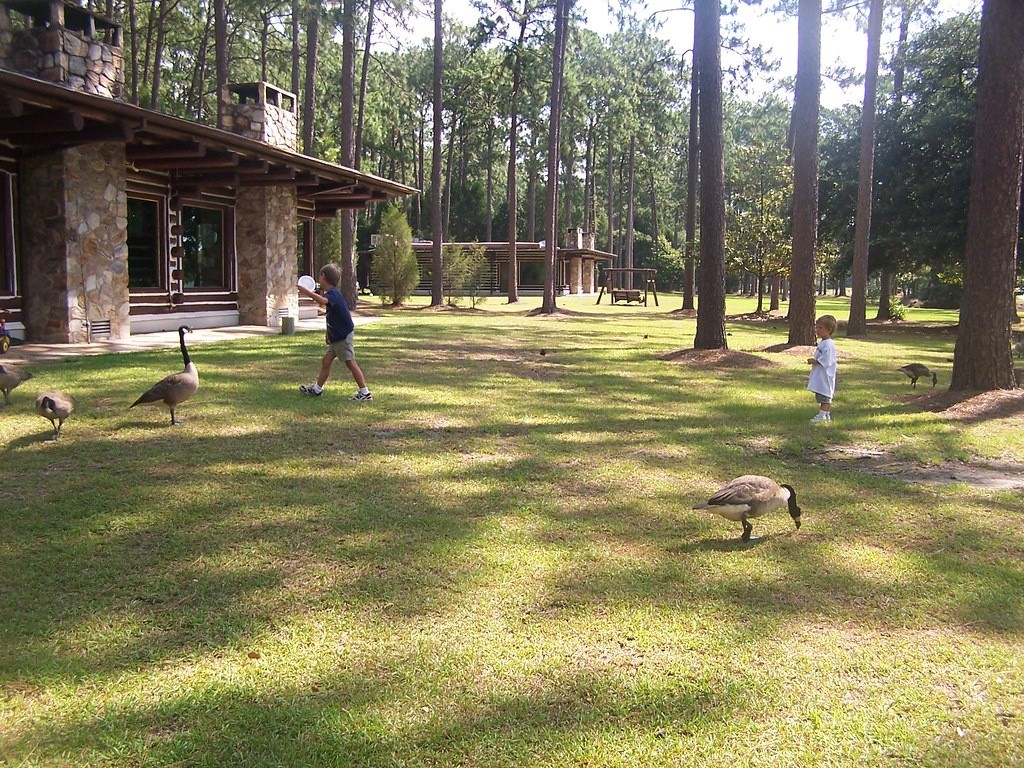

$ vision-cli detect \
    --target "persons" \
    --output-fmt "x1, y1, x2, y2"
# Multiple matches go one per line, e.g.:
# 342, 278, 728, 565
294, 263, 373, 402
806, 315, 837, 423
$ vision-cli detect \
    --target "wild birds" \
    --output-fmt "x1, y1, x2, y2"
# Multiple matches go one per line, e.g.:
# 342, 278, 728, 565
130, 325, 201, 426
0, 363, 37, 406
897, 362, 937, 390
34, 388, 75, 446
690, 474, 804, 541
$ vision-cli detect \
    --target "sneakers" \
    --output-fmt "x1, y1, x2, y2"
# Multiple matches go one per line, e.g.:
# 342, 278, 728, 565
347, 390, 373, 402
299, 384, 323, 397
810, 415, 831, 424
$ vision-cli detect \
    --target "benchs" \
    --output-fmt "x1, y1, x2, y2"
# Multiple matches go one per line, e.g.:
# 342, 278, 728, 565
612, 288, 646, 305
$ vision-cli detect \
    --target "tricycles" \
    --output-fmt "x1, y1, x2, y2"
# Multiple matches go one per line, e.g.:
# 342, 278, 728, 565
0, 308, 12, 353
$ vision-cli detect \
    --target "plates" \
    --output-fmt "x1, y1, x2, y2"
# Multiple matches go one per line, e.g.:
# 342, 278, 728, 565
298, 275, 315, 292
